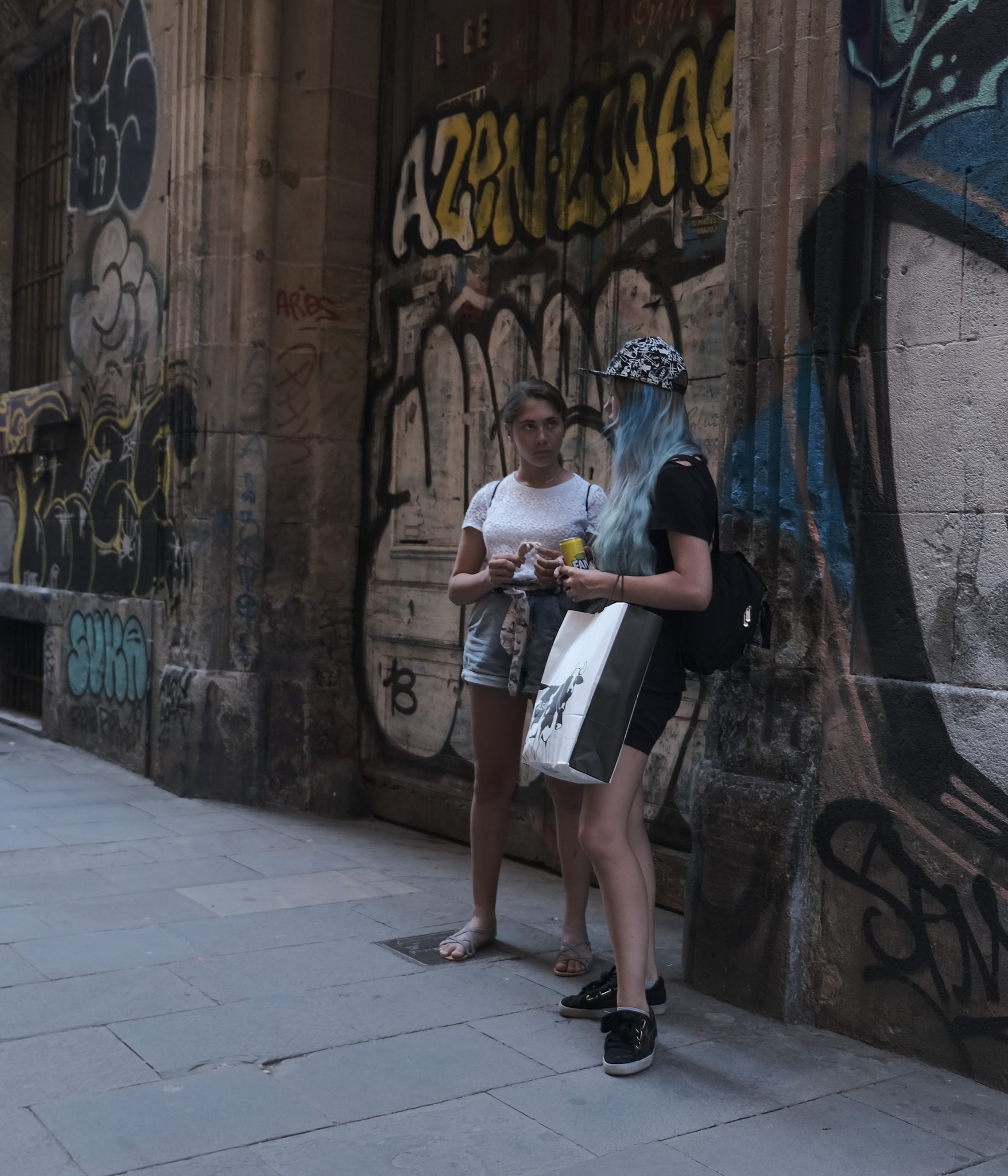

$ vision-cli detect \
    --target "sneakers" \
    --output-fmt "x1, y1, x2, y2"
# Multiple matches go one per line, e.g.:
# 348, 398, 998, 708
599, 1008, 661, 1074
558, 962, 668, 1018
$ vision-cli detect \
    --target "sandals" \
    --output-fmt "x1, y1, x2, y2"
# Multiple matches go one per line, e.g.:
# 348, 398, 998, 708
553, 936, 593, 976
438, 921, 498, 961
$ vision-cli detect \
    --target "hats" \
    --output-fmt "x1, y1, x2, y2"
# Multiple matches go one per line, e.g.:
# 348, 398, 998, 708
578, 334, 688, 395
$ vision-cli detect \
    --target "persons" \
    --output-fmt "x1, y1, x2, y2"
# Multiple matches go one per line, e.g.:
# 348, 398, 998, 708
439, 378, 611, 977
553, 336, 767, 1077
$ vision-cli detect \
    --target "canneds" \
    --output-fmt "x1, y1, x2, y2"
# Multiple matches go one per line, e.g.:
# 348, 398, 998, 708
557, 535, 590, 578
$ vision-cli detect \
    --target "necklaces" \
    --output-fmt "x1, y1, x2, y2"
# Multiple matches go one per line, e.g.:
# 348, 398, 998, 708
518, 466, 563, 485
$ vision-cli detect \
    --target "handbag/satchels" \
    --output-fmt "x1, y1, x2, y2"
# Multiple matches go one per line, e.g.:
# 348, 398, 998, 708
520, 573, 664, 786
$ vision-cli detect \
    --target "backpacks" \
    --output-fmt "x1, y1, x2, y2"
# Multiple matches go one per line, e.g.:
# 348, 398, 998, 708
646, 455, 766, 678
